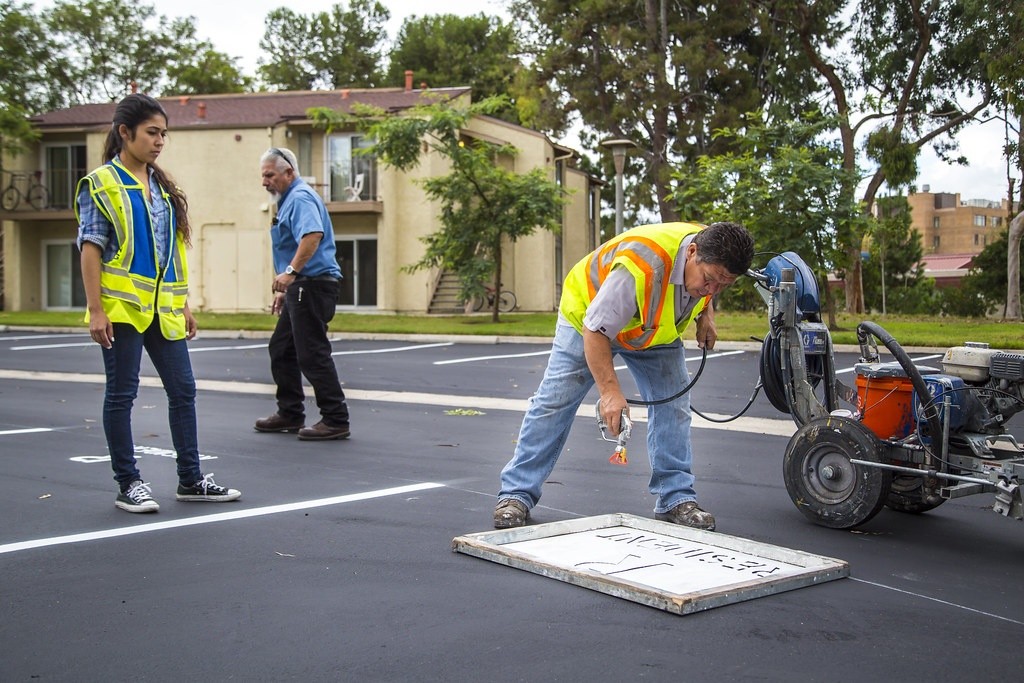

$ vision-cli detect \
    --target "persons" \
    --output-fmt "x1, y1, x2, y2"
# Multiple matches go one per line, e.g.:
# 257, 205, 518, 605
494, 220, 756, 530
253, 147, 352, 442
74, 94, 242, 514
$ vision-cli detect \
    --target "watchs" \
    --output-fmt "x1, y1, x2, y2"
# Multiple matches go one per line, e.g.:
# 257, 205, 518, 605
286, 266, 299, 276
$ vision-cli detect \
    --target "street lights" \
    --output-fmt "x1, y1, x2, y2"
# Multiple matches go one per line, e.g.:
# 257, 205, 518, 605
599, 140, 639, 236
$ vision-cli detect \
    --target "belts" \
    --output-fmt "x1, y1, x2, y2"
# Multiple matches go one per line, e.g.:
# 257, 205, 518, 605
295, 275, 338, 283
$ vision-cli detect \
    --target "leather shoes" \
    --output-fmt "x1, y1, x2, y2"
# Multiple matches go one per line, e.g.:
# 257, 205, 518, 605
298, 420, 350, 440
254, 412, 306, 433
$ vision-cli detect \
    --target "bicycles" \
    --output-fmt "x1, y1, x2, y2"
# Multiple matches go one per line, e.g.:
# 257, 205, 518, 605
473, 278, 518, 313
0, 167, 52, 213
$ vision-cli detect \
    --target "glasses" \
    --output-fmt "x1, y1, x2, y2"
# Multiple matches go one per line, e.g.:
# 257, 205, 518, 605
269, 148, 294, 171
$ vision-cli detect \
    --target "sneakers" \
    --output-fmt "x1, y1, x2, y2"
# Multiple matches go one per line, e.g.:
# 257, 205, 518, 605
115, 481, 159, 512
176, 473, 241, 501
493, 498, 530, 529
655, 502, 716, 530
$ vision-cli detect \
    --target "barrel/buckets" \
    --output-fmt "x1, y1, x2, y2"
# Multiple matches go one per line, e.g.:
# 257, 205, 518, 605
855, 374, 913, 465
